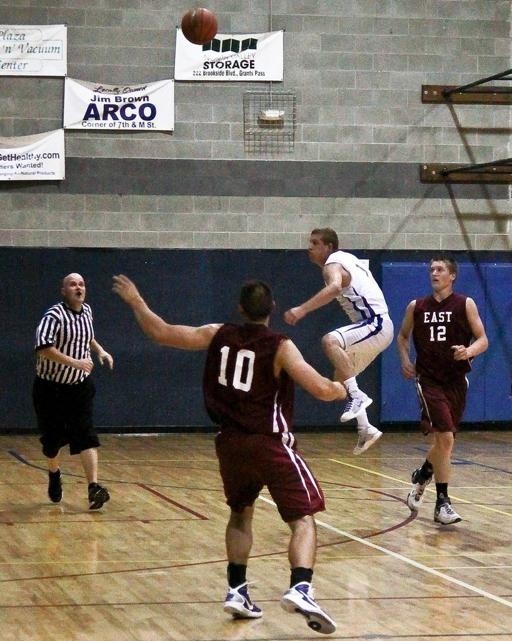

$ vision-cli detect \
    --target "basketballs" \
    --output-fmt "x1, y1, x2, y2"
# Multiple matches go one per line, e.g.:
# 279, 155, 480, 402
182, 6, 219, 45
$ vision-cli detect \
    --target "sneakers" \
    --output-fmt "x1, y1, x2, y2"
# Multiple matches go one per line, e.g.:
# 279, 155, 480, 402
340, 389, 373, 422
353, 424, 383, 455
280, 581, 337, 634
433, 498, 463, 526
87, 487, 111, 511
406, 468, 425, 512
48, 468, 63, 505
222, 580, 262, 619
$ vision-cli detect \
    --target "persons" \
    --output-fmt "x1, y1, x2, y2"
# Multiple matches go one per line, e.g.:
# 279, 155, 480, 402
396, 255, 490, 526
31, 272, 115, 510
282, 227, 396, 456
111, 272, 349, 632
